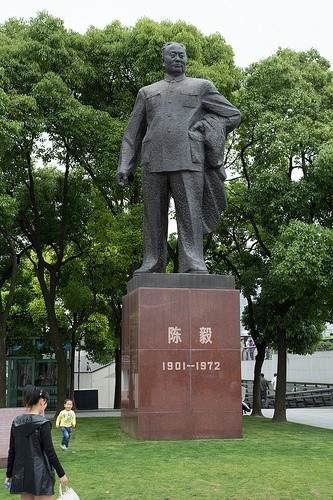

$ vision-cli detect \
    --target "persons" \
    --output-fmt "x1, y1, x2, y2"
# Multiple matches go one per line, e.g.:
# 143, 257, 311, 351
240, 339, 270, 360
259, 373, 268, 407
117, 42, 243, 276
271, 373, 277, 391
55, 399, 76, 450
5, 385, 67, 500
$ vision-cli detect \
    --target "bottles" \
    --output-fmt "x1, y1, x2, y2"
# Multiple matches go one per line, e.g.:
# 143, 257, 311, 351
5, 482, 11, 489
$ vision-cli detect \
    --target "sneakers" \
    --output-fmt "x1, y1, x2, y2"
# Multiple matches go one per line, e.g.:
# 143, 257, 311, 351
59, 443, 68, 450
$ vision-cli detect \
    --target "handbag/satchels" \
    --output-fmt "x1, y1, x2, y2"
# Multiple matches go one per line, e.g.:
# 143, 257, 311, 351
56, 482, 80, 500
